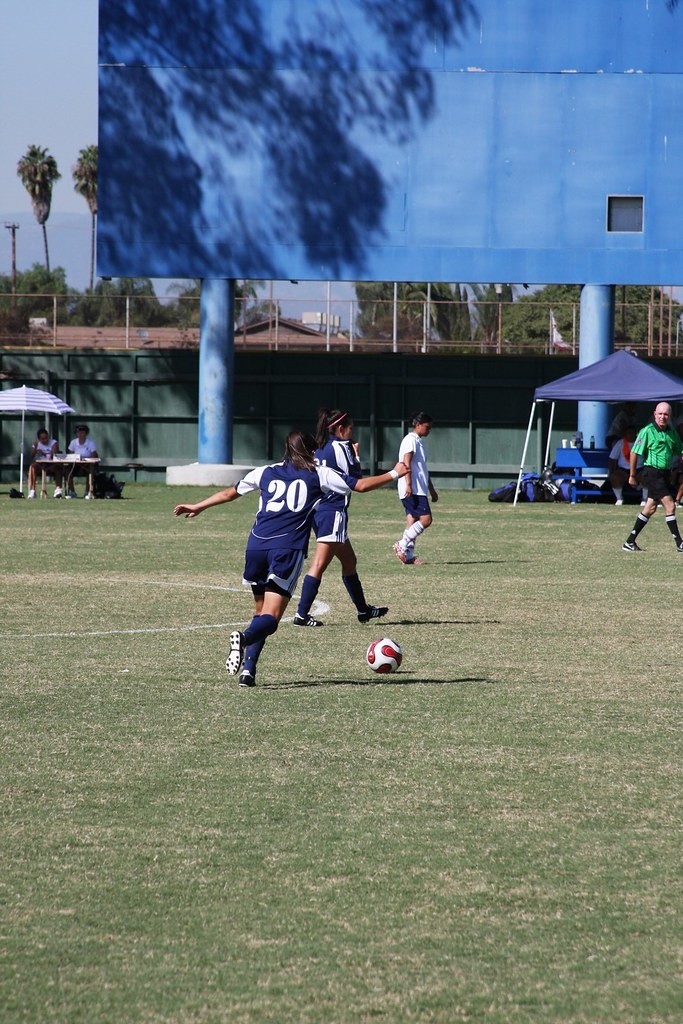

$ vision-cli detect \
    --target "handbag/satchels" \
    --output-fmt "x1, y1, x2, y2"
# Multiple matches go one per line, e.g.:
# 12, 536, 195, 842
93, 471, 126, 499
488, 461, 599, 503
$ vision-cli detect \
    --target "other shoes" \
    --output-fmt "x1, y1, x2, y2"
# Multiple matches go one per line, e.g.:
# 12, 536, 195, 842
54, 489, 63, 498
66, 491, 77, 499
84, 490, 89, 498
27, 490, 37, 498
393, 540, 430, 565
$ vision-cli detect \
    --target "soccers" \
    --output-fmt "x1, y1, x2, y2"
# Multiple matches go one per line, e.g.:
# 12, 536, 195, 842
366, 637, 403, 673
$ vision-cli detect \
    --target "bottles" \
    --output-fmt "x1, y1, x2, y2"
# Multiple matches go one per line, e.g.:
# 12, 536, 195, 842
589, 435, 595, 450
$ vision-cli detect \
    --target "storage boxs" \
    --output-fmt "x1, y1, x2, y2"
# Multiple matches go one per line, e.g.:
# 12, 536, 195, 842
53, 453, 81, 461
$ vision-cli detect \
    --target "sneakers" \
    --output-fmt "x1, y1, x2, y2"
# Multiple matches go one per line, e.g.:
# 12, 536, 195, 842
676, 541, 683, 553
357, 606, 388, 624
225, 631, 245, 676
622, 541, 641, 551
238, 670, 257, 687
293, 612, 324, 627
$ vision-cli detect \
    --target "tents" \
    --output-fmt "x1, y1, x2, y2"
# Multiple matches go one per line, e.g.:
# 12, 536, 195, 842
508, 344, 682, 507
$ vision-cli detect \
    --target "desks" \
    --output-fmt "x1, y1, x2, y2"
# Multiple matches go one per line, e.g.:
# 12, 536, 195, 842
36, 461, 98, 501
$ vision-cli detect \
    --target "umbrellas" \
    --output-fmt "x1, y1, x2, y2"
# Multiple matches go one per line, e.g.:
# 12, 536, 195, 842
1, 385, 77, 496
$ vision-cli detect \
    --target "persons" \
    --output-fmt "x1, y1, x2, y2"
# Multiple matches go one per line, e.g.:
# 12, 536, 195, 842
172, 431, 410, 684
391, 411, 439, 566
23, 428, 64, 500
63, 424, 99, 500
292, 401, 413, 627
602, 401, 683, 553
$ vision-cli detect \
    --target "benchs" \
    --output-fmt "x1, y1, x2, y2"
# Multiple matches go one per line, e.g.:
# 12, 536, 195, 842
551, 448, 642, 504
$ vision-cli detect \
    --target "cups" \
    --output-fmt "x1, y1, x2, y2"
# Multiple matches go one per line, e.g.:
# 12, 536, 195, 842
562, 439, 567, 448
576, 432, 583, 450
570, 440, 577, 449
